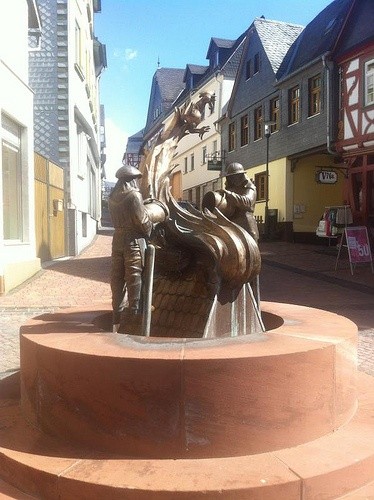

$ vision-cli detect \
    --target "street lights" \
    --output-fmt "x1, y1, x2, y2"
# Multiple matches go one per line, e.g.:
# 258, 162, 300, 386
260, 121, 277, 237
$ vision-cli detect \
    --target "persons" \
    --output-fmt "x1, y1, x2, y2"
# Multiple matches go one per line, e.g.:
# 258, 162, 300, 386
108, 166, 153, 324
215, 162, 260, 310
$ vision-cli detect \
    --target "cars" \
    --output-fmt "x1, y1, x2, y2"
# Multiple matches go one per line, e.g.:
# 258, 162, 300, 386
316, 205, 343, 242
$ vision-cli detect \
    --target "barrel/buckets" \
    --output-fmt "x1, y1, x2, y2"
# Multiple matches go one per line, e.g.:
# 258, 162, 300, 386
201, 190, 237, 219
144, 198, 170, 229
